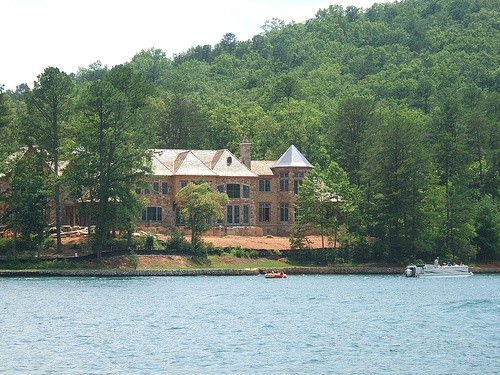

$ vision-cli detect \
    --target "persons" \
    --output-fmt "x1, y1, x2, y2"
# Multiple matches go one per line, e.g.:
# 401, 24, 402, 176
447, 262, 452, 266
459, 261, 464, 266
434, 257, 439, 268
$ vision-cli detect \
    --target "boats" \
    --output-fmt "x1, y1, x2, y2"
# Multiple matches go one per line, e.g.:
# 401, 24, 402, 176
399, 262, 475, 280
